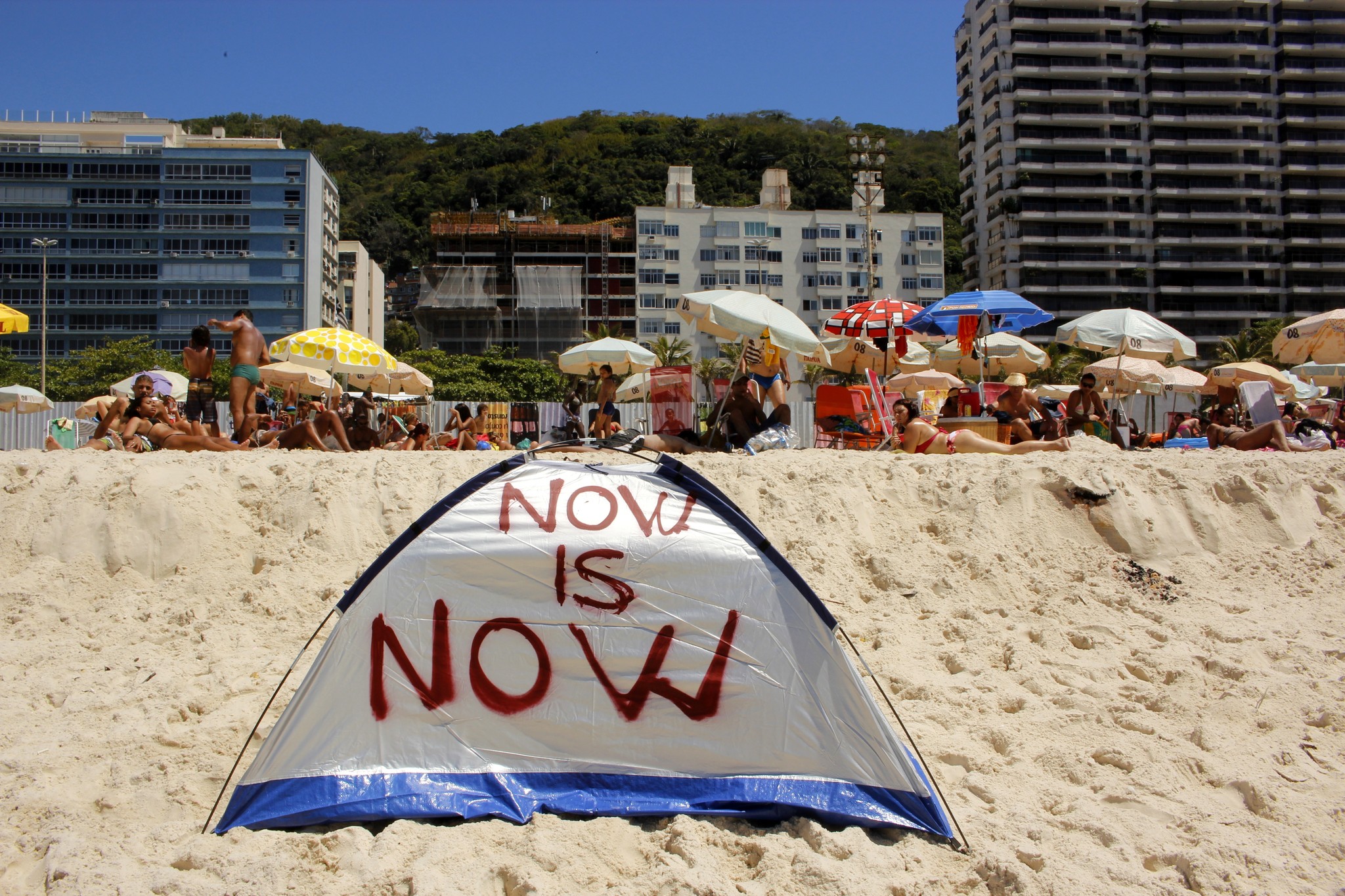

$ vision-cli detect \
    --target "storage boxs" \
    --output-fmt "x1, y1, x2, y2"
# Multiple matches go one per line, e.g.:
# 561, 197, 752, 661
936, 415, 998, 442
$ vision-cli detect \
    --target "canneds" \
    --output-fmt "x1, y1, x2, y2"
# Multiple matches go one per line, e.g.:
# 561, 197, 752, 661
748, 430, 790, 452
965, 405, 971, 417
745, 445, 757, 456
724, 443, 731, 453
735, 449, 746, 454
957, 401, 965, 417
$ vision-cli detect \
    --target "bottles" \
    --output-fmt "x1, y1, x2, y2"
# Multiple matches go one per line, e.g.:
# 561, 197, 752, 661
744, 443, 757, 456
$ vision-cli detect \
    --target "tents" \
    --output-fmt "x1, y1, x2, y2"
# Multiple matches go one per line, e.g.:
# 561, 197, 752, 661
202, 436, 970, 848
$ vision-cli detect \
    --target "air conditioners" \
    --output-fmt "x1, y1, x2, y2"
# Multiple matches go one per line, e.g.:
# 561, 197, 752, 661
324, 276, 337, 302
161, 301, 170, 308
872, 265, 878, 269
324, 219, 330, 224
726, 286, 731, 290
857, 288, 864, 292
333, 230, 337, 235
704, 286, 710, 290
289, 201, 295, 206
858, 264, 863, 268
169, 252, 177, 258
649, 236, 655, 240
205, 251, 215, 257
287, 301, 294, 306
72, 198, 80, 204
3, 274, 12, 279
287, 251, 295, 258
290, 177, 295, 181
149, 198, 157, 204
238, 251, 247, 258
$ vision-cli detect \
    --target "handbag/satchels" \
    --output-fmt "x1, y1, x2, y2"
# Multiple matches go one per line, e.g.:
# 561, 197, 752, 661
1299, 430, 1331, 448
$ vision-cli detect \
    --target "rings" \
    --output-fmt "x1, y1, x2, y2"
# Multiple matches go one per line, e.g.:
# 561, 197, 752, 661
133, 442, 134, 443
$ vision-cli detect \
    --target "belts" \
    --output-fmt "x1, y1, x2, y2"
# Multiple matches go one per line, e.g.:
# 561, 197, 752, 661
189, 378, 212, 382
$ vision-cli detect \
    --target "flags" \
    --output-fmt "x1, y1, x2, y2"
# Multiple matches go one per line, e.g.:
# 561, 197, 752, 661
334, 297, 349, 330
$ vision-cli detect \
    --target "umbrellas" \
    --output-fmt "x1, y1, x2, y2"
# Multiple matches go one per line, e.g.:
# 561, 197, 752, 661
0, 304, 29, 335
75, 394, 117, 420
43, 418, 98, 438
348, 361, 434, 444
613, 369, 689, 428
110, 366, 190, 402
558, 336, 663, 376
813, 288, 1345, 440
267, 325, 398, 411
258, 361, 343, 425
675, 290, 832, 448
0, 384, 54, 449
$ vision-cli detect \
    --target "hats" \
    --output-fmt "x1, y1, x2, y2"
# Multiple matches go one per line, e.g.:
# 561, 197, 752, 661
949, 389, 960, 397
730, 371, 750, 382
1004, 373, 1027, 386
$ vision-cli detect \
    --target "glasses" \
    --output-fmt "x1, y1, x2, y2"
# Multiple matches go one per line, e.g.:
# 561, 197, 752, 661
414, 417, 418, 420
1082, 382, 1095, 388
1342, 409, 1345, 412
360, 422, 368, 425
490, 436, 497, 442
425, 433, 430, 436
165, 395, 170, 399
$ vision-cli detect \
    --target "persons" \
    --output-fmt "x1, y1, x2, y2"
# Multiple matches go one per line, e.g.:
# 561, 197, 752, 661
44, 364, 791, 454
740, 337, 790, 425
208, 309, 271, 433
891, 371, 1345, 455
183, 324, 220, 437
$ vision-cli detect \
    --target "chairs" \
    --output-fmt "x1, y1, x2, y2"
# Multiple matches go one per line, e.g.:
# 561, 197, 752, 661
1036, 397, 1073, 438
1238, 379, 1345, 446
391, 413, 453, 450
712, 376, 760, 444
978, 380, 1008, 415
46, 417, 80, 448
587, 407, 621, 439
255, 394, 285, 431
1161, 410, 1191, 447
813, 366, 942, 451
635, 361, 699, 435
